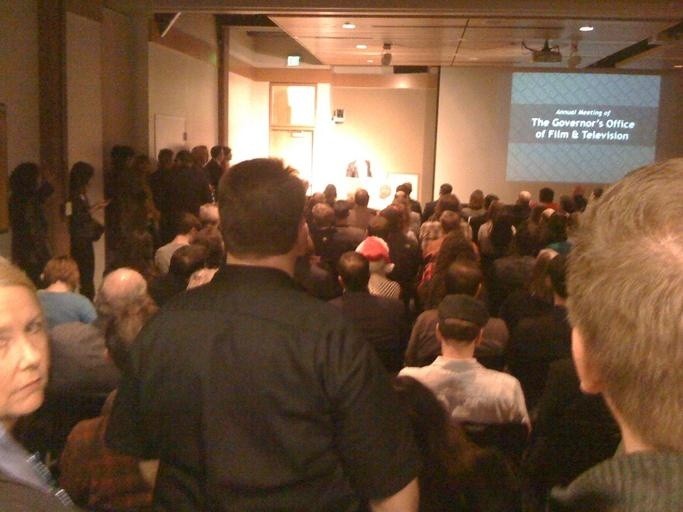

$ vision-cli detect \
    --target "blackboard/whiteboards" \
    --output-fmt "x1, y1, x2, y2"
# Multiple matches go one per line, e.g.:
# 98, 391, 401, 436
155, 114, 187, 161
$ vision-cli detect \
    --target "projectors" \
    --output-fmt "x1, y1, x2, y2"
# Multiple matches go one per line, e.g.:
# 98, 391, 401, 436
533, 50, 561, 62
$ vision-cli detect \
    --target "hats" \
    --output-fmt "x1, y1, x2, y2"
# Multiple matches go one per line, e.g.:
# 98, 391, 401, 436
356, 237, 395, 273
438, 295, 489, 326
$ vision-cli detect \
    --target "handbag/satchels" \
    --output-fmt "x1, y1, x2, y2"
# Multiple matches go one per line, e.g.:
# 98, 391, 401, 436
69, 211, 104, 241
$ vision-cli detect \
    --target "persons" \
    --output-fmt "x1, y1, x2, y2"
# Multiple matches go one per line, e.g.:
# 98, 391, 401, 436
1, 145, 683, 510
